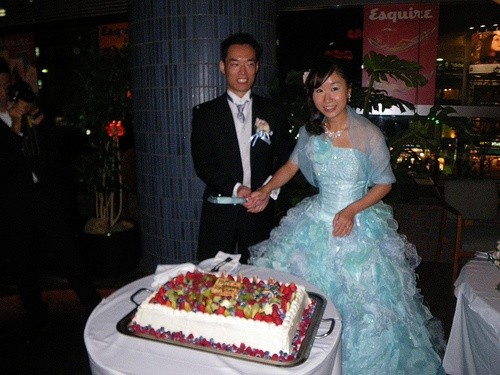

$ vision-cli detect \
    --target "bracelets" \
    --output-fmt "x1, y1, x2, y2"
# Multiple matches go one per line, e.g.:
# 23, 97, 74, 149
32, 110, 42, 120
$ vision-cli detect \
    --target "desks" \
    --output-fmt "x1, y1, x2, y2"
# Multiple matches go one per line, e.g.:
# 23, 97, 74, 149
83, 262, 342, 375
440, 260, 500, 375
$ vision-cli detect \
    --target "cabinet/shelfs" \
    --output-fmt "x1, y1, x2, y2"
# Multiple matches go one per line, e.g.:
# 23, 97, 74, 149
460, 24, 500, 173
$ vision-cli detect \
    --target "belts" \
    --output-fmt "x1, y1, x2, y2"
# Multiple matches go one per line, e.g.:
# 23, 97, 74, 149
208, 196, 249, 205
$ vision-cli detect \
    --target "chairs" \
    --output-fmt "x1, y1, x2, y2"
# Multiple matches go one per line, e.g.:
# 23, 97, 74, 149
439, 180, 500, 298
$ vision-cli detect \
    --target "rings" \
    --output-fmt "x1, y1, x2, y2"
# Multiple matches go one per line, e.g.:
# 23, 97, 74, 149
346, 225, 349, 228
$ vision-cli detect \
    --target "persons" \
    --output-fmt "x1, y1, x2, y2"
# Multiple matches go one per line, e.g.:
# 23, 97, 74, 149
0, 56, 102, 333
190, 33, 292, 264
250, 59, 445, 375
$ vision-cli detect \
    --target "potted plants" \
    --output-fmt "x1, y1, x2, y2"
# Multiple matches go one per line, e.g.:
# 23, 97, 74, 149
71, 44, 140, 275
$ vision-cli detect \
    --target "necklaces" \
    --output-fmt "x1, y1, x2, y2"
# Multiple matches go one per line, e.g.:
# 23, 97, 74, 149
323, 118, 347, 141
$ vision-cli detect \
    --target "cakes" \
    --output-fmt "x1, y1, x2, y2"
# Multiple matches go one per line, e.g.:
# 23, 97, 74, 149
127, 268, 317, 362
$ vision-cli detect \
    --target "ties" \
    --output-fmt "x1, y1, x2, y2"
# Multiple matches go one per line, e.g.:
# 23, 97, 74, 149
237, 101, 248, 123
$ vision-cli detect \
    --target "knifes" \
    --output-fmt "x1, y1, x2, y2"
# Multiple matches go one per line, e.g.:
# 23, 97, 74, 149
207, 196, 247, 205
